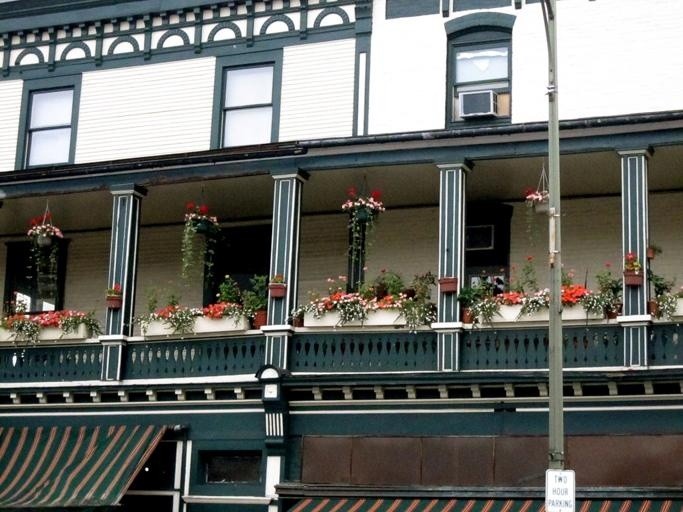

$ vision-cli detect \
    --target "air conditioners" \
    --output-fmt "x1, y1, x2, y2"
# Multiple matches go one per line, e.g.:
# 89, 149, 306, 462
459, 90, 498, 117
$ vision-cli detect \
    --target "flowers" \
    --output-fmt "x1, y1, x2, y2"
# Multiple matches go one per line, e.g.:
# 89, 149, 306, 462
0, 187, 683, 361
